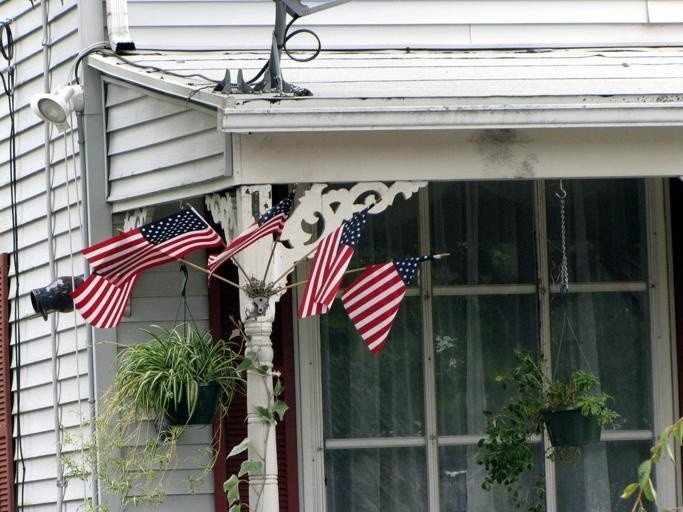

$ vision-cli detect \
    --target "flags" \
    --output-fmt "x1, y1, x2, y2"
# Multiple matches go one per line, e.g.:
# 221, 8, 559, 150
66, 264, 139, 333
205, 197, 288, 283
295, 207, 371, 318
82, 207, 220, 289
338, 253, 430, 354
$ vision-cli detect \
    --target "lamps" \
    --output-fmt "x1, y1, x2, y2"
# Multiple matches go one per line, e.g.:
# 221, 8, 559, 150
30, 84, 84, 126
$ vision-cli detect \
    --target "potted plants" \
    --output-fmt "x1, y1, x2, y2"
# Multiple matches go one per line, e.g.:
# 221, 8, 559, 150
470, 347, 622, 512
59, 320, 264, 512
